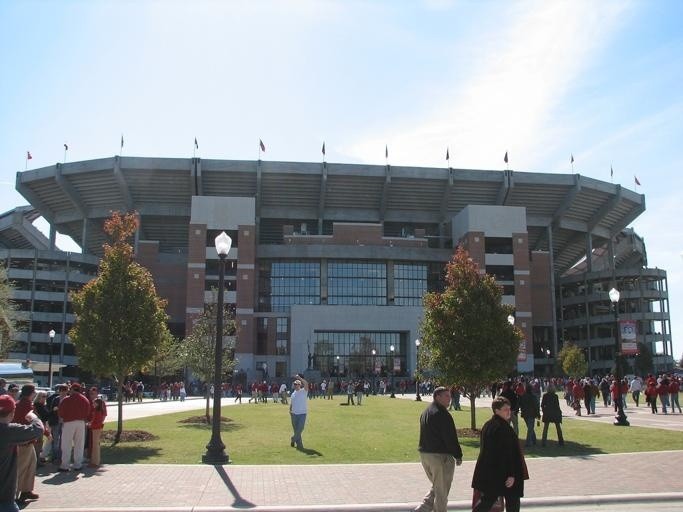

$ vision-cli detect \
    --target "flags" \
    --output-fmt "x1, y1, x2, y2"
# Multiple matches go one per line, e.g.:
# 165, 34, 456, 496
609, 165, 614, 177
258, 139, 266, 153
384, 144, 389, 159
444, 147, 450, 161
62, 144, 69, 152
502, 151, 509, 164
26, 151, 32, 160
569, 153, 574, 165
320, 141, 326, 157
193, 137, 199, 150
119, 134, 124, 149
632, 175, 642, 188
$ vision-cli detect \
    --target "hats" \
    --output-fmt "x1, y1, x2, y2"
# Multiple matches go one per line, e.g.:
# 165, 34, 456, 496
8, 384, 19, 391
293, 380, 301, 384
0, 394, 17, 414
69, 383, 81, 389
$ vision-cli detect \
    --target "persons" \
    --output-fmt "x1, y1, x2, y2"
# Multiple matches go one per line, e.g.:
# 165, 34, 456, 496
470, 396, 529, 512
0, 377, 187, 511
410, 386, 462, 511
539, 384, 564, 448
498, 381, 518, 438
201, 371, 683, 417
514, 383, 541, 445
286, 374, 309, 451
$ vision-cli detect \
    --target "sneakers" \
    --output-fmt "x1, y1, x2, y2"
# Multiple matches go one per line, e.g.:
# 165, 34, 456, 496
291, 441, 304, 450
40, 457, 97, 473
14, 499, 25, 507
20, 491, 39, 500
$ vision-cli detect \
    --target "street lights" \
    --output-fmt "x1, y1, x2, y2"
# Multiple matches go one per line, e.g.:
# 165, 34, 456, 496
192, 231, 234, 464
414, 339, 423, 401
607, 287, 629, 426
369, 349, 378, 395
388, 344, 397, 398
46, 328, 55, 388
507, 314, 516, 328
336, 355, 340, 381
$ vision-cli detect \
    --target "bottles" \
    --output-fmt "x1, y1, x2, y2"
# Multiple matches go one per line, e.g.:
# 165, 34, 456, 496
537, 417, 540, 427
